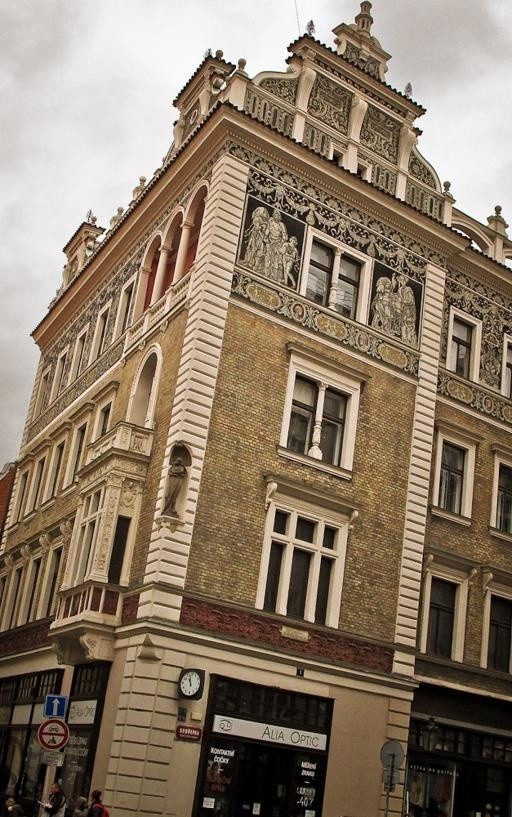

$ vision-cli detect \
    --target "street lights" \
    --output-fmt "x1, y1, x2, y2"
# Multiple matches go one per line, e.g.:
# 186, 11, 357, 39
420, 717, 440, 816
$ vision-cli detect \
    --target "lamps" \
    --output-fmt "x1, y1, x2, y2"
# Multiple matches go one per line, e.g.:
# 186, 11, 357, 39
422, 713, 440, 752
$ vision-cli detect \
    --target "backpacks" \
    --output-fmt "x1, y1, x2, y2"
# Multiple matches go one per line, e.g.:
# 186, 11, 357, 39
92, 804, 110, 817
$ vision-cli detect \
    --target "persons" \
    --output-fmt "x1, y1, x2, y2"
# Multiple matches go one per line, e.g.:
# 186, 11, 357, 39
0, 782, 104, 817
161, 456, 188, 517
371, 271, 417, 345
240, 207, 300, 290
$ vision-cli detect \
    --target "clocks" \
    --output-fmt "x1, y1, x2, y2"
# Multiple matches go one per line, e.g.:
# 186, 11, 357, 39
178, 669, 205, 700
